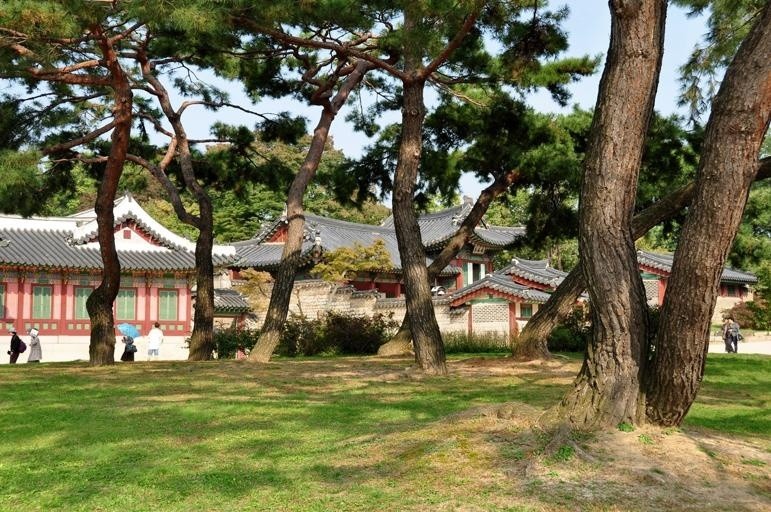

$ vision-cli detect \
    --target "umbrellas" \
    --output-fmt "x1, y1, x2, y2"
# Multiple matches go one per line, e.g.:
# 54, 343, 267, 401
117, 323, 139, 338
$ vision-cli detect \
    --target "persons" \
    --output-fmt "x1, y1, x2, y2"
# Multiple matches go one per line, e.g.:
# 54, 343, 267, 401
8, 328, 20, 364
728, 317, 739, 352
722, 318, 733, 353
27, 328, 42, 363
121, 336, 134, 361
147, 322, 163, 360
